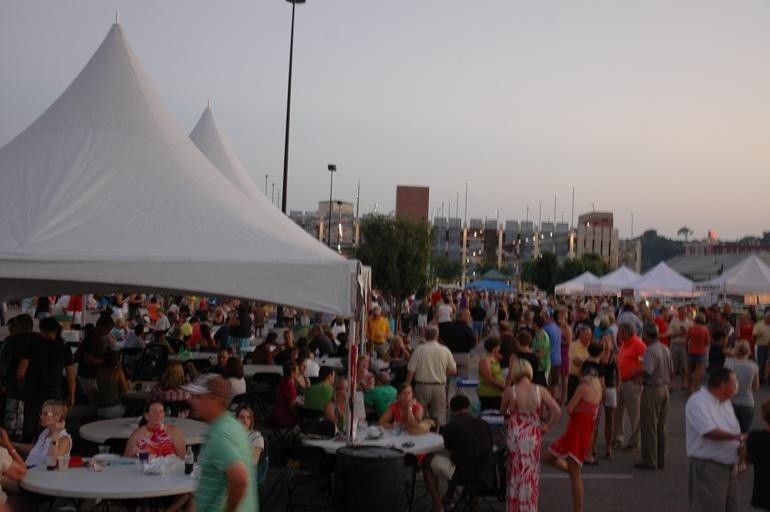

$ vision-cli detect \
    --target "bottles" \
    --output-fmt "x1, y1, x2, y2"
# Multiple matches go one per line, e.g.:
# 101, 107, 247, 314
184, 446, 194, 476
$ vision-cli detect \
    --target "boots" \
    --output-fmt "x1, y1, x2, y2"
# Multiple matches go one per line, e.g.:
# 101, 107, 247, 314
613, 440, 657, 470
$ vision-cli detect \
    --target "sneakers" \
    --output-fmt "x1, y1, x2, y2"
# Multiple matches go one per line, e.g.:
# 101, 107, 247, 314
592, 449, 615, 462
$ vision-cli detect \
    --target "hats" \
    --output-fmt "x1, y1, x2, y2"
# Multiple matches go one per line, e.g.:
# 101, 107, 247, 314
158, 307, 165, 312
372, 306, 382, 314
181, 374, 230, 399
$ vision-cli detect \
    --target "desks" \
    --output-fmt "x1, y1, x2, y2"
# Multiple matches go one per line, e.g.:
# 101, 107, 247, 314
0, 291, 508, 511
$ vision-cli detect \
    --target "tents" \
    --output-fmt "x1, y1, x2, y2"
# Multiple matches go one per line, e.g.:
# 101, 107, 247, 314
691, 253, 770, 308
555, 261, 704, 307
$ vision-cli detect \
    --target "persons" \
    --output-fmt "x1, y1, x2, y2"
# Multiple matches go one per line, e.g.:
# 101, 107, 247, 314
550, 298, 769, 511
402, 288, 561, 511
0, 287, 413, 511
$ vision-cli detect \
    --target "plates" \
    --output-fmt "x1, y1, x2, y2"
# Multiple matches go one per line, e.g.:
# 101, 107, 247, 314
94, 454, 121, 461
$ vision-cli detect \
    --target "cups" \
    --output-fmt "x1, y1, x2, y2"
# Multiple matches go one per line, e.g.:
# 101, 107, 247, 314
99, 446, 109, 454
137, 450, 149, 464
393, 421, 402, 436
46, 455, 57, 471
57, 456, 72, 471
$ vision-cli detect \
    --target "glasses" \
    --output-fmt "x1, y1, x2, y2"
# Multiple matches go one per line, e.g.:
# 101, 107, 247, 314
38, 411, 56, 417
